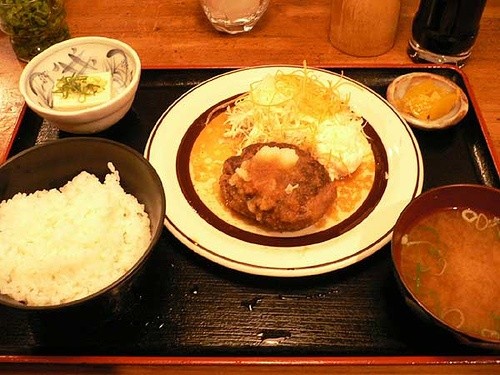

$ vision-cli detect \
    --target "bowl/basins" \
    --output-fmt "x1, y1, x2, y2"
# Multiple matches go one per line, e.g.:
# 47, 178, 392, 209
391, 183, 500, 349
19, 36, 141, 134
0, 137, 166, 311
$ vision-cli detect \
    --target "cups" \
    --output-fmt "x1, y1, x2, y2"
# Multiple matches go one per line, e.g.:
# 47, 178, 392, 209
407, 0, 486, 69
0, 0, 70, 64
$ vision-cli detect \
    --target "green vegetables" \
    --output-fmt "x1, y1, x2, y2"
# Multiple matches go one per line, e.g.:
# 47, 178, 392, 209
0, 0, 72, 67
52, 72, 96, 102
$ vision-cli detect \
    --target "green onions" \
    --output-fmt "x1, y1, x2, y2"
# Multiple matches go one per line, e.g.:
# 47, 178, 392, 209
401, 224, 500, 341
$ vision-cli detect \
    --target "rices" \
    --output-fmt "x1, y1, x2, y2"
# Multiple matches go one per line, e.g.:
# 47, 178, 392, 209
0, 161, 151, 306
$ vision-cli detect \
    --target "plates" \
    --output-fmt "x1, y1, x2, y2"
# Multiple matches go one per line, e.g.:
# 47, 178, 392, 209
143, 64, 424, 278
200, 0, 270, 34
